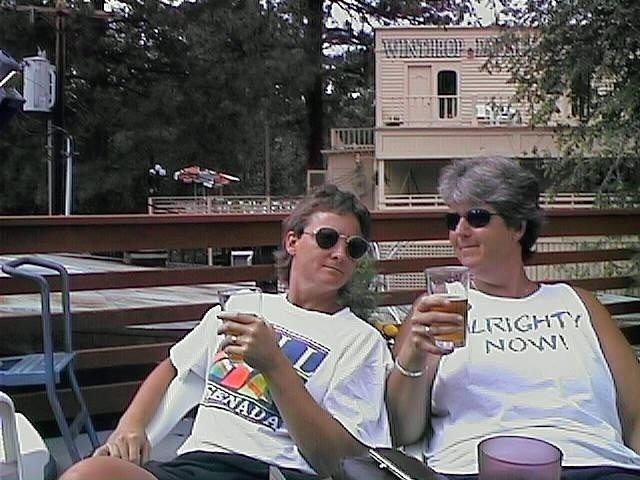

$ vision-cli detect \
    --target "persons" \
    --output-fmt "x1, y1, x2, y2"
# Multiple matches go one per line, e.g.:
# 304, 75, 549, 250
61, 184, 392, 480
384, 156, 640, 480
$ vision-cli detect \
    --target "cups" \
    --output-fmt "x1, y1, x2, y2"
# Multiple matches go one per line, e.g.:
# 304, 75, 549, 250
425, 265, 469, 348
477, 435, 562, 479
217, 288, 263, 365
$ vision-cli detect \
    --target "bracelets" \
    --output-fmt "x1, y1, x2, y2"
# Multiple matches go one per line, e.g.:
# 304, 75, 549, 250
394, 355, 429, 379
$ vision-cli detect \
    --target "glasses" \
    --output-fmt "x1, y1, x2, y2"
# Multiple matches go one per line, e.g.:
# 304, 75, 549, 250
304, 225, 370, 261
445, 208, 502, 232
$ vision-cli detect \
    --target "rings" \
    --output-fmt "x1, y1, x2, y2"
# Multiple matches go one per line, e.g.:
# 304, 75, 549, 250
425, 326, 432, 335
231, 334, 236, 342
